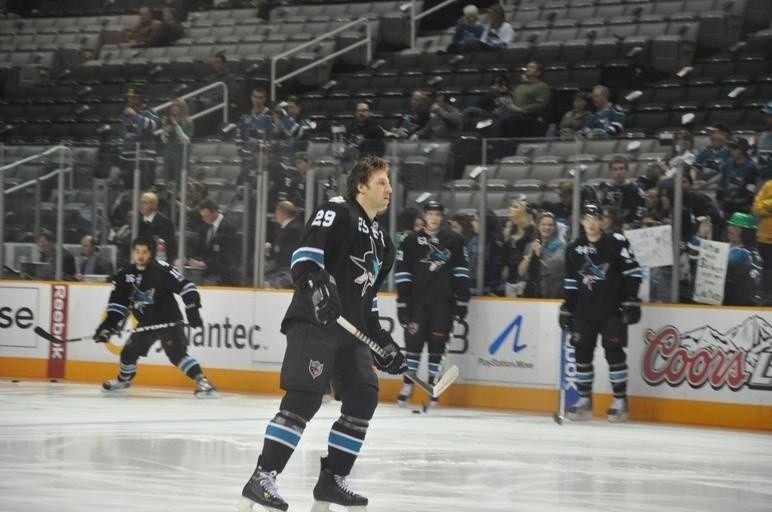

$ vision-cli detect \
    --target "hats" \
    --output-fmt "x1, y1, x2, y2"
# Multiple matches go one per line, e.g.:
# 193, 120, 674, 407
356, 103, 369, 111
552, 181, 574, 194
726, 212, 758, 229
626, 47, 644, 58
705, 124, 748, 150
729, 41, 746, 52
760, 101, 772, 115
127, 82, 146, 97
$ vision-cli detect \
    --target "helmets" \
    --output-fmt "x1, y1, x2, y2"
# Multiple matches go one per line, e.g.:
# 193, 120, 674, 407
423, 200, 443, 213
583, 203, 602, 218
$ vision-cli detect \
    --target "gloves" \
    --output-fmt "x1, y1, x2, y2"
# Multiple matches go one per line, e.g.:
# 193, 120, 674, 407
94, 312, 125, 343
187, 309, 202, 329
307, 271, 342, 327
397, 302, 409, 329
622, 296, 641, 324
456, 301, 468, 320
560, 304, 574, 329
371, 332, 408, 374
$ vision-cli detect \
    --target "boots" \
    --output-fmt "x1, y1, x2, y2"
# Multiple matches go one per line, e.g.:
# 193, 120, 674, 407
103, 371, 136, 390
314, 457, 368, 506
194, 377, 215, 395
242, 455, 288, 512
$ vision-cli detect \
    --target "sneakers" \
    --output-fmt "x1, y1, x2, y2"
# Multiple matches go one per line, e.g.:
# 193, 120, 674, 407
570, 397, 592, 413
398, 383, 438, 401
607, 398, 628, 415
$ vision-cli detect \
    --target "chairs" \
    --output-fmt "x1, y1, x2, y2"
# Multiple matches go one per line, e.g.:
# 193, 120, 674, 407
0, 0, 772, 280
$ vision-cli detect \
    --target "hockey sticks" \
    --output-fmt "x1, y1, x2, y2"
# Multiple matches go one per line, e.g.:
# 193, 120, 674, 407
337, 316, 458, 397
34, 318, 177, 343
553, 331, 566, 423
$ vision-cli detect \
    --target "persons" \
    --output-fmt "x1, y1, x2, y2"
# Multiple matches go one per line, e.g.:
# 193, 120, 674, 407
240, 155, 408, 512
1, 0, 771, 304
392, 200, 471, 408
558, 203, 645, 422
91, 237, 221, 399
